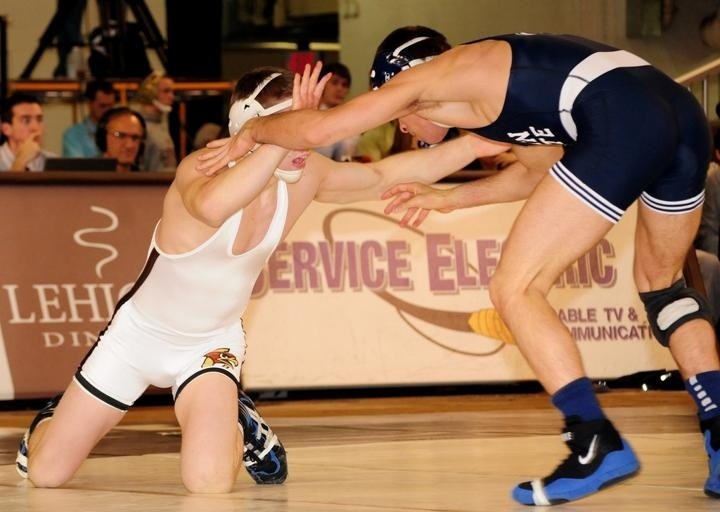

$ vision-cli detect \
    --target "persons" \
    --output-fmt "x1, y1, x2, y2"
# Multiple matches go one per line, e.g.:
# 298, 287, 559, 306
10, 61, 509, 496
692, 12, 719, 315
194, 24, 719, 505
0, 62, 517, 175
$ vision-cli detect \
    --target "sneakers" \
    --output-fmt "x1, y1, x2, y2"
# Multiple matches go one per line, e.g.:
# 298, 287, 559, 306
698, 410, 720, 497
512, 415, 641, 506
15, 391, 66, 479
237, 386, 288, 485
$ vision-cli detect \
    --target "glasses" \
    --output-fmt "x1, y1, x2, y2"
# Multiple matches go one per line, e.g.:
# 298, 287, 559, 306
99, 123, 143, 141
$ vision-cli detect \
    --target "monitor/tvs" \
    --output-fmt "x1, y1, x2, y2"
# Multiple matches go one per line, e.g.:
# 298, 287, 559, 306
42, 156, 118, 174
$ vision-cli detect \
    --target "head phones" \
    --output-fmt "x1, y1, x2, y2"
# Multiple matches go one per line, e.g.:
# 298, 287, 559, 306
95, 106, 146, 156
